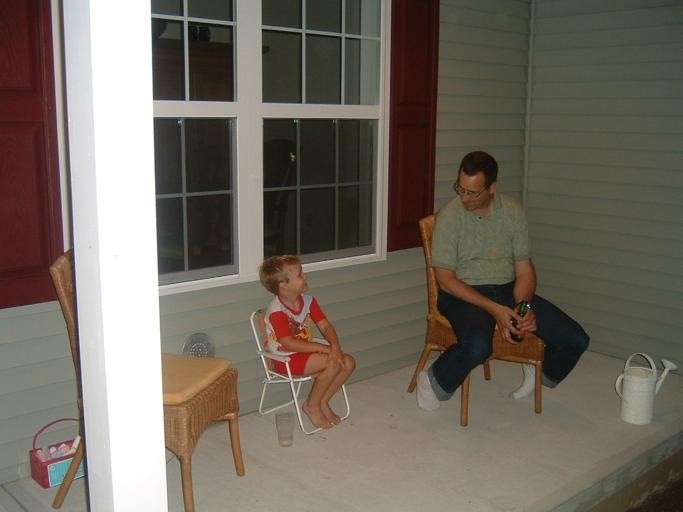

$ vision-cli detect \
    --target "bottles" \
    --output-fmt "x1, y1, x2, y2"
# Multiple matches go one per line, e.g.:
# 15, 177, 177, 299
510, 300, 527, 342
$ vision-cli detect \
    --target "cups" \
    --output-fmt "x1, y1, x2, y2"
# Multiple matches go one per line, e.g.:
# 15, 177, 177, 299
274, 413, 294, 447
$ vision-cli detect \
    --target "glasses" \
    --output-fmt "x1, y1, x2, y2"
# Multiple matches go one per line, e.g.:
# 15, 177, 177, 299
454, 180, 488, 197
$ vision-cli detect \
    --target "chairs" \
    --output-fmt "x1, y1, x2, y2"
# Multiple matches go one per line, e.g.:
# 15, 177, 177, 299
407, 211, 546, 427
247, 308, 353, 434
46, 247, 245, 511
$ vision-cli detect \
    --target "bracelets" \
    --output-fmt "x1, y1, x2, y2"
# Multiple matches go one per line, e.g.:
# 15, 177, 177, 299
514, 300, 528, 307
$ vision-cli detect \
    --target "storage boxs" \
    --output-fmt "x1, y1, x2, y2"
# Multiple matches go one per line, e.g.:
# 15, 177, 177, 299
26, 417, 84, 489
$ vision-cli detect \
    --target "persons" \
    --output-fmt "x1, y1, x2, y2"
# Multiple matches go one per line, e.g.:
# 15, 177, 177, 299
259, 254, 355, 430
416, 150, 590, 412
